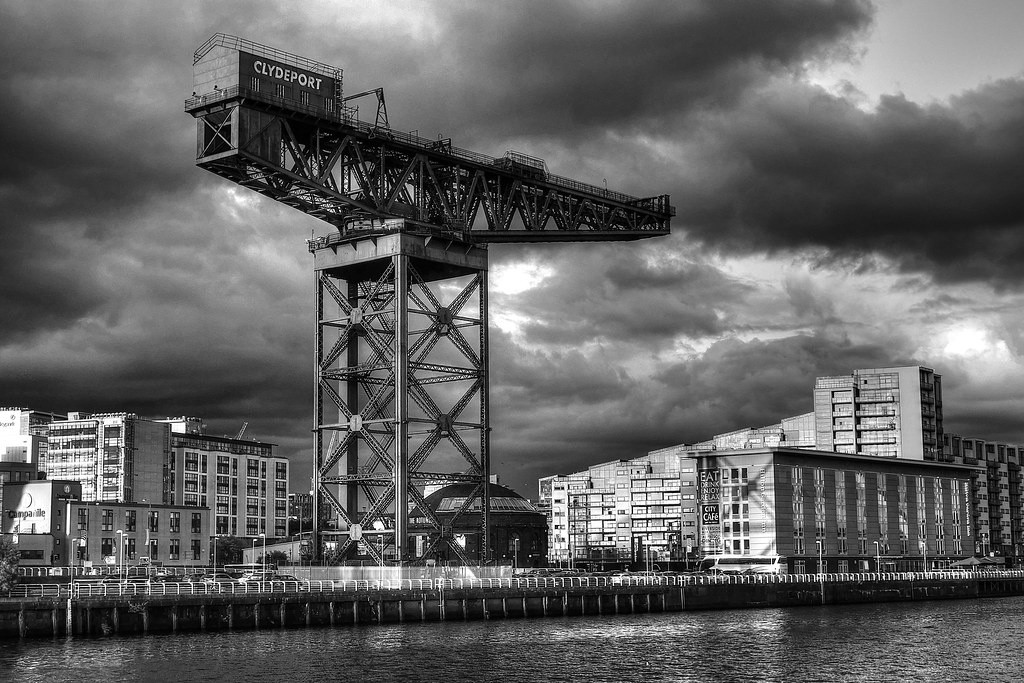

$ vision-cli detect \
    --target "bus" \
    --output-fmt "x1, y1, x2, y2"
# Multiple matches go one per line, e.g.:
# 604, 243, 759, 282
696, 555, 788, 575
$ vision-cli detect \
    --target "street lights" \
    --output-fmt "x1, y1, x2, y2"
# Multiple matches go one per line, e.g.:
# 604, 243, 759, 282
514, 538, 520, 568
770, 539, 774, 575
148, 539, 158, 595
378, 534, 385, 590
252, 539, 258, 574
816, 540, 822, 581
70, 538, 77, 600
293, 534, 298, 576
213, 538, 220, 591
920, 542, 928, 574
115, 530, 123, 596
142, 498, 152, 532
122, 533, 129, 588
259, 533, 266, 593
572, 499, 579, 567
873, 540, 880, 574
295, 506, 303, 566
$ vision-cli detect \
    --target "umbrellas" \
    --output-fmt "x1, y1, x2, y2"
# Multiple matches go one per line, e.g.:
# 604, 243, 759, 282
950, 557, 995, 571
